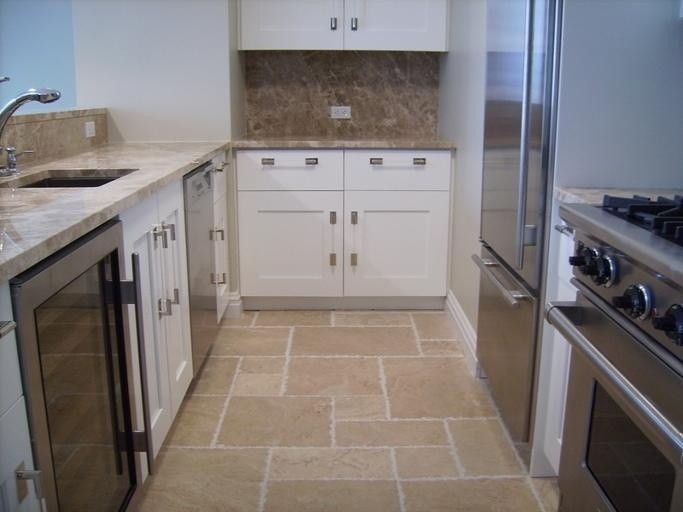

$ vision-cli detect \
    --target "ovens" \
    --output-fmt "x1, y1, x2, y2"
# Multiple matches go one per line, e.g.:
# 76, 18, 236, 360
541, 277, 682, 510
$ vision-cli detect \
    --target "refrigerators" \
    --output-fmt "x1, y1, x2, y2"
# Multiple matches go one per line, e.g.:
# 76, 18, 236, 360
473, 3, 682, 483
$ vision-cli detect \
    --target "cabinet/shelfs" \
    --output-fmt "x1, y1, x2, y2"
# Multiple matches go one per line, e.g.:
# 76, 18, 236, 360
0, 280, 50, 511
235, 1, 451, 53
235, 147, 451, 311
213, 153, 232, 323
119, 174, 196, 483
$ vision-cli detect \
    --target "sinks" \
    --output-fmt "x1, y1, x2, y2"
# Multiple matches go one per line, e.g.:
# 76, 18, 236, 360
0, 164, 140, 190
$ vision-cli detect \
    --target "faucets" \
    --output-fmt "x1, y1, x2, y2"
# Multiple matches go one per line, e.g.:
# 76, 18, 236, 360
0, 86, 61, 179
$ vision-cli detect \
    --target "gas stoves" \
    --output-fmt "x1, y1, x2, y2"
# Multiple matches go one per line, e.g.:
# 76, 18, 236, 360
600, 188, 682, 245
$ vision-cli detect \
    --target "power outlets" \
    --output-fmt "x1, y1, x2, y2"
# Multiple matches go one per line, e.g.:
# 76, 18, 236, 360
331, 105, 351, 119
